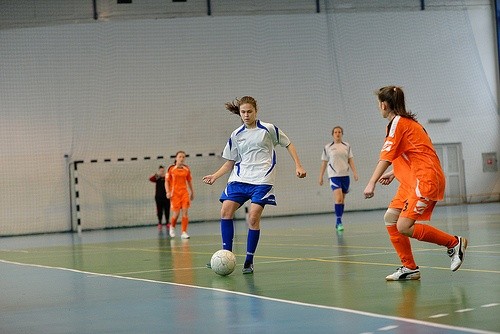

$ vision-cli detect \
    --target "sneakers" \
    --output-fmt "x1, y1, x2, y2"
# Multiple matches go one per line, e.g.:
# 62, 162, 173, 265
385, 266, 421, 280
206, 263, 211, 268
242, 261, 254, 274
446, 236, 467, 271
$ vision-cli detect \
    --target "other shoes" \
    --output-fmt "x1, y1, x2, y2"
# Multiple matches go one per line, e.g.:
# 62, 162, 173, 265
181, 232, 190, 239
159, 224, 162, 228
169, 225, 175, 238
166, 223, 171, 228
337, 224, 344, 231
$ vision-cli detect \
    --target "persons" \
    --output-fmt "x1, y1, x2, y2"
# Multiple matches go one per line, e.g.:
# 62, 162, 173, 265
149, 165, 170, 231
202, 96, 306, 274
363, 86, 467, 281
319, 126, 358, 231
165, 151, 194, 239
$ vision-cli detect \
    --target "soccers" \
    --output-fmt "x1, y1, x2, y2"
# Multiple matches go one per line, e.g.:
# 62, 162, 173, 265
209, 249, 236, 276
